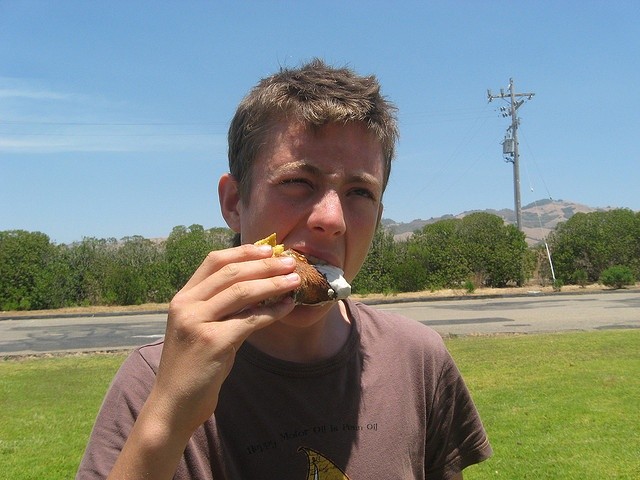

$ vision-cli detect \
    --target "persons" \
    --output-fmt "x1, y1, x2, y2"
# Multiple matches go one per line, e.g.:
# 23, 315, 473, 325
74, 58, 492, 480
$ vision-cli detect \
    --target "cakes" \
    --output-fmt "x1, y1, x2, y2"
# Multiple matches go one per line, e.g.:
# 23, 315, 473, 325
247, 233, 352, 309
